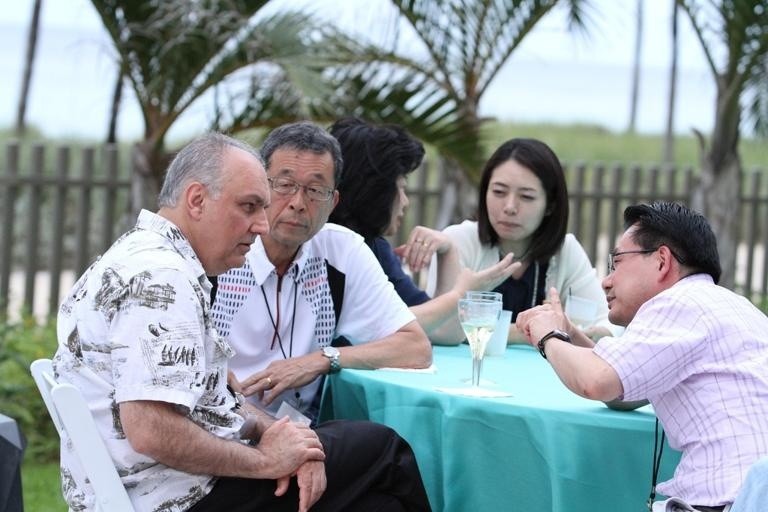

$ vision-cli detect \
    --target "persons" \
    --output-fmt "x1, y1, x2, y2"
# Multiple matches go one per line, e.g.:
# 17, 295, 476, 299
207, 121, 432, 446
326, 116, 523, 347
515, 199, 768, 512
48, 129, 432, 512
407, 136, 626, 345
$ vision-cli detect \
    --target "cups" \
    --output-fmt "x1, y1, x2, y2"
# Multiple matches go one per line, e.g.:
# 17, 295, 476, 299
486, 308, 512, 356
564, 292, 598, 337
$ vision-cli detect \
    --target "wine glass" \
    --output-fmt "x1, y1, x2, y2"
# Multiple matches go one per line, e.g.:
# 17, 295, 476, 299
458, 289, 502, 398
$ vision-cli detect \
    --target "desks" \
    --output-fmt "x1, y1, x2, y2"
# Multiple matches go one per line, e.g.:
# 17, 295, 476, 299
324, 340, 682, 511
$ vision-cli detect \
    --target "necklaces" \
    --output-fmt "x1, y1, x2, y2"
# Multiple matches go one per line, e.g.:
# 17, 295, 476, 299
500, 241, 540, 307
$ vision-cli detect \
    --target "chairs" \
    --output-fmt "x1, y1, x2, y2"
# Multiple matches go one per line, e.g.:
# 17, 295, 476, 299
30, 359, 137, 511
728, 459, 767, 512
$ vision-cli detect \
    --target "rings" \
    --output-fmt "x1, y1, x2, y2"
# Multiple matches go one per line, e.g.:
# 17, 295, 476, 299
415, 239, 423, 243
267, 377, 274, 388
421, 241, 429, 247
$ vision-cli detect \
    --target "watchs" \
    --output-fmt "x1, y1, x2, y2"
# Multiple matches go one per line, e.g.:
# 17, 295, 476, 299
537, 329, 571, 359
320, 346, 341, 375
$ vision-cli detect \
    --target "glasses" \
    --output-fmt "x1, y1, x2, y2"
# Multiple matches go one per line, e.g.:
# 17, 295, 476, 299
268, 177, 336, 204
604, 248, 685, 274
